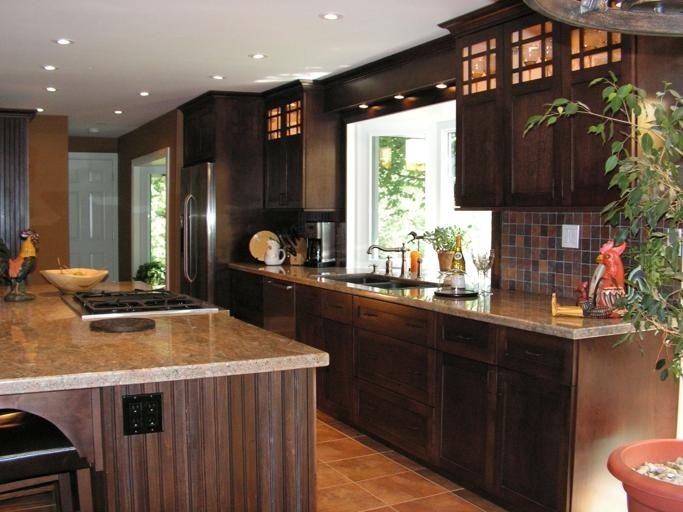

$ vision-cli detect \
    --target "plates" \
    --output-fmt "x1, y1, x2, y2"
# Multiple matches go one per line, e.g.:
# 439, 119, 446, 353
248, 231, 280, 261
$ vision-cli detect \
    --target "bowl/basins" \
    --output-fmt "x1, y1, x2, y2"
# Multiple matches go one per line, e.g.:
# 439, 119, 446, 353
38, 266, 110, 293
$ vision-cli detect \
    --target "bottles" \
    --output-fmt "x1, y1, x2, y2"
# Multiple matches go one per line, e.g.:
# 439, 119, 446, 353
416, 258, 421, 278
410, 243, 421, 274
450, 234, 465, 288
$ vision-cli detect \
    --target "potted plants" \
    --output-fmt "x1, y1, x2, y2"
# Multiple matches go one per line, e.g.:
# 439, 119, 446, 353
421, 225, 472, 271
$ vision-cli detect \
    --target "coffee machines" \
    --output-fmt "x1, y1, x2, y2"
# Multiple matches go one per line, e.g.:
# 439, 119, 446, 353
303, 221, 336, 267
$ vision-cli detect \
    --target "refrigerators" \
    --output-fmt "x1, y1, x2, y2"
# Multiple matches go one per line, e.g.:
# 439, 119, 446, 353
178, 160, 215, 303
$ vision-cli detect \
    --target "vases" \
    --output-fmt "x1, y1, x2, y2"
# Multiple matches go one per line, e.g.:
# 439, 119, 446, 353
604, 437, 682, 511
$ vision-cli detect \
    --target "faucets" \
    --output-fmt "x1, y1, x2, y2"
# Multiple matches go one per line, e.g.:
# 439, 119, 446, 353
367, 243, 410, 278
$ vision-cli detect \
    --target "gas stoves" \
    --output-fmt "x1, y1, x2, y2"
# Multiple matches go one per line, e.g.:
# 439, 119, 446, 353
74, 287, 205, 315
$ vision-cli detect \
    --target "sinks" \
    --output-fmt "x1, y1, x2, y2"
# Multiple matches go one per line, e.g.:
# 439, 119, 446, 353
313, 273, 406, 285
363, 282, 450, 291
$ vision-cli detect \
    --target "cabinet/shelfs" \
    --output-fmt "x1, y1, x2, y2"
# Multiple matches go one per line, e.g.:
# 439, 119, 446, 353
510, 12, 563, 210
294, 285, 354, 428
260, 78, 342, 214
436, 311, 679, 512
226, 271, 262, 328
564, 1, 682, 211
351, 296, 438, 472
457, 20, 511, 210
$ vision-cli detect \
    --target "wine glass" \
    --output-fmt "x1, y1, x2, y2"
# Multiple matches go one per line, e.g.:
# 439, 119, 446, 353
471, 246, 496, 296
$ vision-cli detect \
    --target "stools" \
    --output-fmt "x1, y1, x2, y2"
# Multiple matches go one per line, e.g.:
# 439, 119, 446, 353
0, 411, 95, 511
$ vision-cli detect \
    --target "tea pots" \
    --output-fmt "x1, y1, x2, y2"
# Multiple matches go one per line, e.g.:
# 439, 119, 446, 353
264, 240, 286, 265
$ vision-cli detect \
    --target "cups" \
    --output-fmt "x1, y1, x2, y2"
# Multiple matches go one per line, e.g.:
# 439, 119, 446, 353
435, 270, 478, 294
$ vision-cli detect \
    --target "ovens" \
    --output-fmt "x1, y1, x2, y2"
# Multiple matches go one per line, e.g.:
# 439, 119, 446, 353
261, 276, 296, 341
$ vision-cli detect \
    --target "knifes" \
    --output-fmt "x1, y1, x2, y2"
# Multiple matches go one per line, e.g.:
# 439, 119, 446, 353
276, 228, 299, 256
